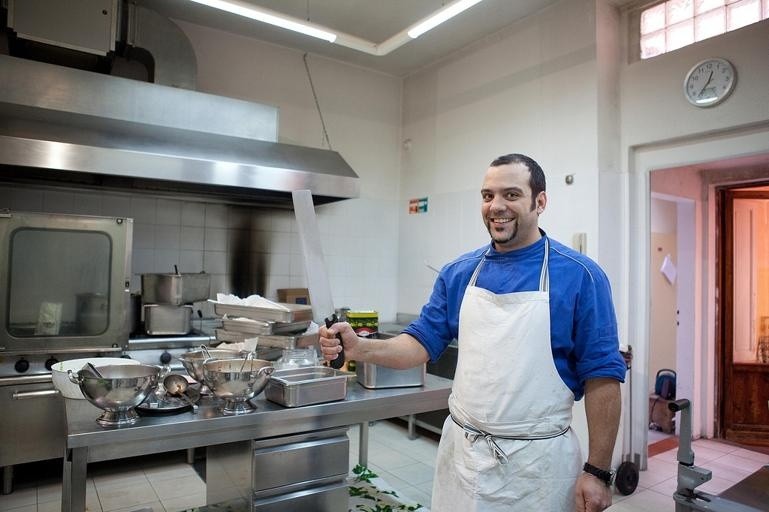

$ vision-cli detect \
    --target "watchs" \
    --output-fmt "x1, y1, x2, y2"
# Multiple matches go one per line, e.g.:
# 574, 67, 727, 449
582, 461, 616, 488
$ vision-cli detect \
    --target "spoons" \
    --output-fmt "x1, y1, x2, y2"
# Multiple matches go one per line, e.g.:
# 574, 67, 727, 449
162, 374, 200, 414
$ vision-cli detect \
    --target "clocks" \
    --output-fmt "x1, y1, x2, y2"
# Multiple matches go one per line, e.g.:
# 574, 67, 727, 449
683, 56, 737, 108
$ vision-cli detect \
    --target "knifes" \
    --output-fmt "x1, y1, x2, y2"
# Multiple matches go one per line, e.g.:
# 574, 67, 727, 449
292, 189, 346, 370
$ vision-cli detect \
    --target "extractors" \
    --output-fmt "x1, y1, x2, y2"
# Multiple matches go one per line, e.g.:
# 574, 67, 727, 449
4, 3, 362, 213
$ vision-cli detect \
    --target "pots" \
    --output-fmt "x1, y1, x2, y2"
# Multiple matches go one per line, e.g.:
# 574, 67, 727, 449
74, 293, 145, 340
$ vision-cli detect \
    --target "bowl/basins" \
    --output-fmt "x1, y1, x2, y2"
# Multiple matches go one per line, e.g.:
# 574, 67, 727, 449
51, 357, 144, 400
174, 349, 248, 395
134, 272, 210, 307
67, 365, 161, 427
203, 358, 275, 415
356, 361, 426, 389
145, 303, 193, 336
265, 365, 358, 408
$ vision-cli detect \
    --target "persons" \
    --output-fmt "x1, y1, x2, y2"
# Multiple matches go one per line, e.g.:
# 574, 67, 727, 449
319, 154, 626, 511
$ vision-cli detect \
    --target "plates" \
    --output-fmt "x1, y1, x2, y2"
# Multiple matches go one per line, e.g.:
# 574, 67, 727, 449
207, 299, 313, 362
136, 386, 201, 414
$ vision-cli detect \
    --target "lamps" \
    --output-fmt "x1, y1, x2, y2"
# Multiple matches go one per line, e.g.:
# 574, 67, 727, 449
191, 0, 337, 43
408, 0, 484, 38
